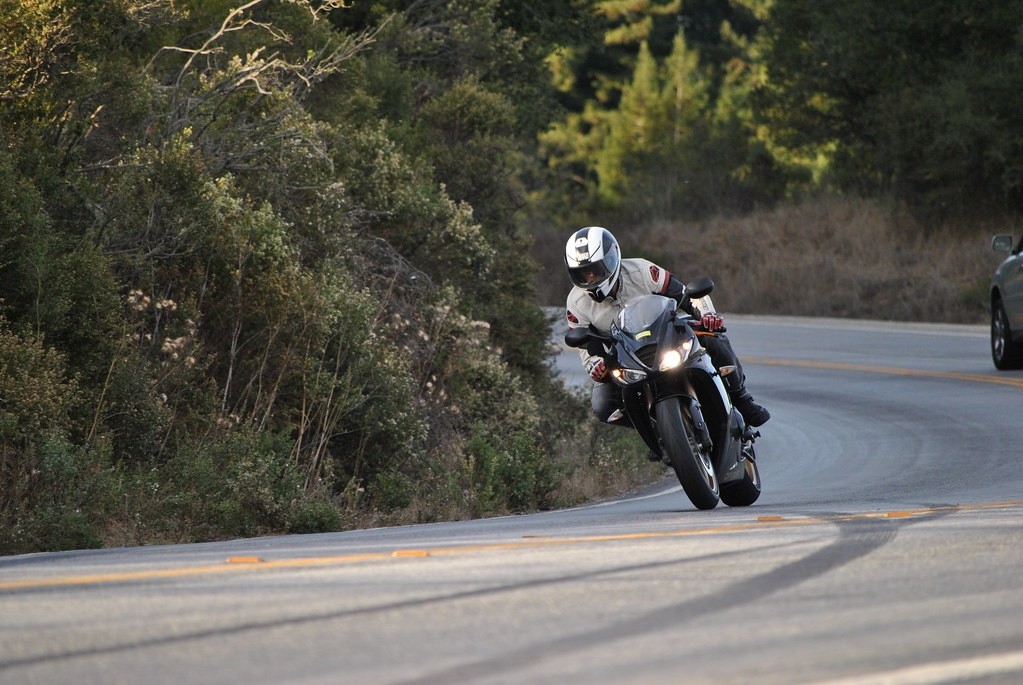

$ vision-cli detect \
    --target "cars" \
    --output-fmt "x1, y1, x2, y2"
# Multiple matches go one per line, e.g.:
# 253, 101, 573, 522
987, 233, 1023, 371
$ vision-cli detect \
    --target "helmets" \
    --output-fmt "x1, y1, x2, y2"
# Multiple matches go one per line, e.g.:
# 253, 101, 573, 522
564, 227, 622, 303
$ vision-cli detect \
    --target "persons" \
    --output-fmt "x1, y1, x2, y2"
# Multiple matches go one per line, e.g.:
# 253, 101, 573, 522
566, 226, 771, 430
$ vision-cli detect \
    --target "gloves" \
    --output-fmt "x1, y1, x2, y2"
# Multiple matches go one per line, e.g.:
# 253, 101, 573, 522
586, 357, 610, 382
702, 312, 724, 332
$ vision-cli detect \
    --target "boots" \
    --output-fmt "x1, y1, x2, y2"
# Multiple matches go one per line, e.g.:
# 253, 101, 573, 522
649, 449, 661, 462
729, 386, 770, 427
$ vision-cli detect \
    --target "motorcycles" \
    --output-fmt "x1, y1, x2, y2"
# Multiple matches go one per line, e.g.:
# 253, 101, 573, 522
564, 277, 762, 510
